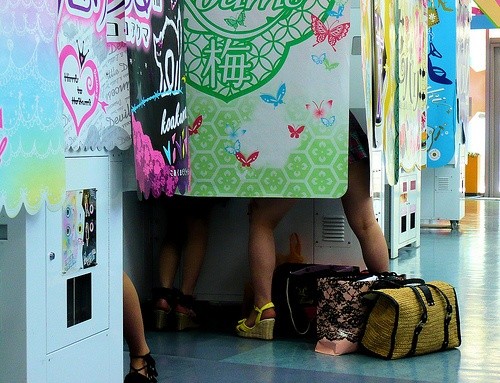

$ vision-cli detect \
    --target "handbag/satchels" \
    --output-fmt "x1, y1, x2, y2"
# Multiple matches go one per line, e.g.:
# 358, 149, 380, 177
272, 263, 461, 359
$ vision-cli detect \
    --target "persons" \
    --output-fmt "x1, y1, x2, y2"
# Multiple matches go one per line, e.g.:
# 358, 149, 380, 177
152, 196, 213, 331
123, 271, 159, 383
233, 111, 390, 340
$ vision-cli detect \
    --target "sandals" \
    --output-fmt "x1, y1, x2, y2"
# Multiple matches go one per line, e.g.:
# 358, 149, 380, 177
123, 352, 157, 383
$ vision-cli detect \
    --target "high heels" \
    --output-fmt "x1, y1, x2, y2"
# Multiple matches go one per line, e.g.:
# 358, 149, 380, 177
235, 301, 274, 340
150, 287, 172, 331
174, 292, 200, 332
